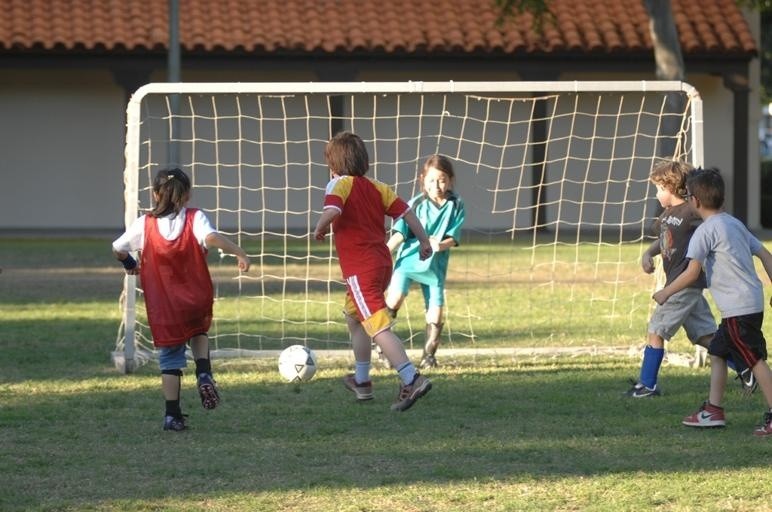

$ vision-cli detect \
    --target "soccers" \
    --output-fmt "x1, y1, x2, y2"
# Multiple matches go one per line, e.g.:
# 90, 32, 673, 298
278, 345, 317, 382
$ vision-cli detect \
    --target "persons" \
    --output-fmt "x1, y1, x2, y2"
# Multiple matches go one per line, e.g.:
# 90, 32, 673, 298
314, 131, 432, 411
620, 156, 759, 399
652, 170, 772, 436
111, 169, 251, 431
377, 154, 465, 369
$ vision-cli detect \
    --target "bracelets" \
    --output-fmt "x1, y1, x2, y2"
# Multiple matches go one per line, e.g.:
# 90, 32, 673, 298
120, 253, 137, 269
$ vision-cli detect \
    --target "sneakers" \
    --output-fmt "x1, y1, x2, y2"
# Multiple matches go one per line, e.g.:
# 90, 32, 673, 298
391, 373, 431, 412
753, 412, 772, 436
623, 382, 661, 398
739, 368, 758, 395
164, 415, 184, 433
682, 400, 726, 426
343, 374, 373, 400
419, 353, 436, 369
198, 372, 219, 409
